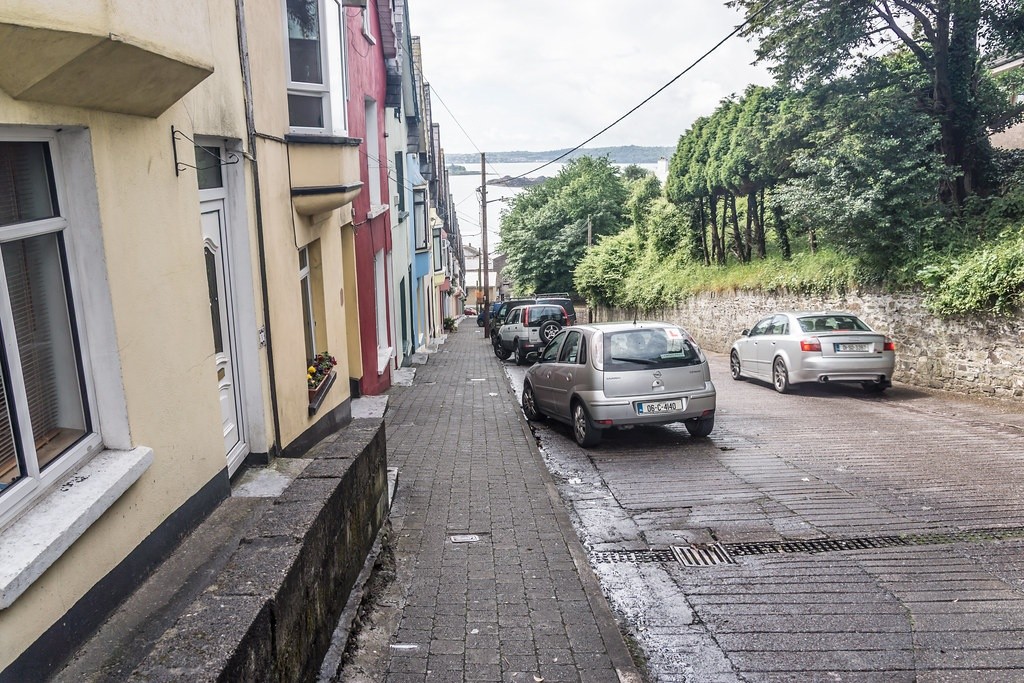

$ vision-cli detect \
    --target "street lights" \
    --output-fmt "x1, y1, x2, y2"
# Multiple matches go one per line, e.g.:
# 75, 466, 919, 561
482, 196, 509, 339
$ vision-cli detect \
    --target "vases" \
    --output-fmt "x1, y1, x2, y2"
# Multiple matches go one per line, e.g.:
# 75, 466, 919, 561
308, 369, 331, 399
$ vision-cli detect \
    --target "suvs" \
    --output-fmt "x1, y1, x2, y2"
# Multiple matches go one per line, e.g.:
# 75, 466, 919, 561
493, 304, 570, 366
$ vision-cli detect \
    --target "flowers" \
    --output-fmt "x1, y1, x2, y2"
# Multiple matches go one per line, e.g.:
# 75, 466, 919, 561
307, 351, 338, 388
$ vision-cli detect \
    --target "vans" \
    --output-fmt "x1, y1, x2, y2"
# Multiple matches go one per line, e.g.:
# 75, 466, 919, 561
477, 299, 577, 338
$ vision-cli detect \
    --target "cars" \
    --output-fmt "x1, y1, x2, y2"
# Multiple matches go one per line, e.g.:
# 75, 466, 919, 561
729, 309, 896, 394
522, 320, 716, 447
